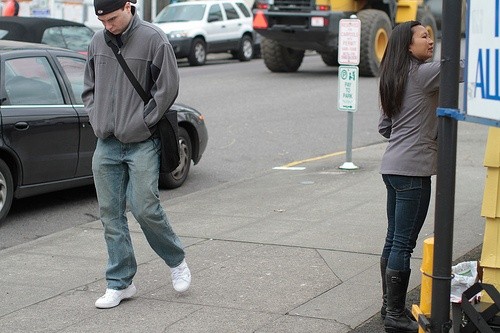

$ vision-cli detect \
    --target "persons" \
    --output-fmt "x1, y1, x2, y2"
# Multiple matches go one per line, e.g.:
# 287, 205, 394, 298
1, 0, 19, 16
377, 21, 465, 333
81, 0, 191, 308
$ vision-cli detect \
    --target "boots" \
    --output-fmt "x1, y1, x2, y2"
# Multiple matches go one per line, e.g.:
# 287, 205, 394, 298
379, 257, 419, 333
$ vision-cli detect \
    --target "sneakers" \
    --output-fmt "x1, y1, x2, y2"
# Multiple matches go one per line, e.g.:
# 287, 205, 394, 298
170, 258, 191, 293
95, 281, 136, 309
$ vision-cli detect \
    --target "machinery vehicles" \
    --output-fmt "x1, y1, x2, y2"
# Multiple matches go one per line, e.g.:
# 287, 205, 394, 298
250, 0, 437, 77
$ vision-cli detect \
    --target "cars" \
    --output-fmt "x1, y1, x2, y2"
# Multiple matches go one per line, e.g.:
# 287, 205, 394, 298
153, 0, 257, 66
0, 38, 209, 225
0, 16, 95, 83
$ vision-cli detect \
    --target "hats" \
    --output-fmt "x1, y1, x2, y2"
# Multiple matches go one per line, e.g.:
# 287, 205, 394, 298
94, 0, 137, 16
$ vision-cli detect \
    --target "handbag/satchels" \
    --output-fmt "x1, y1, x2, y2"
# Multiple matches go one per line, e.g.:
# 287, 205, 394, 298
449, 261, 483, 303
157, 110, 180, 173
